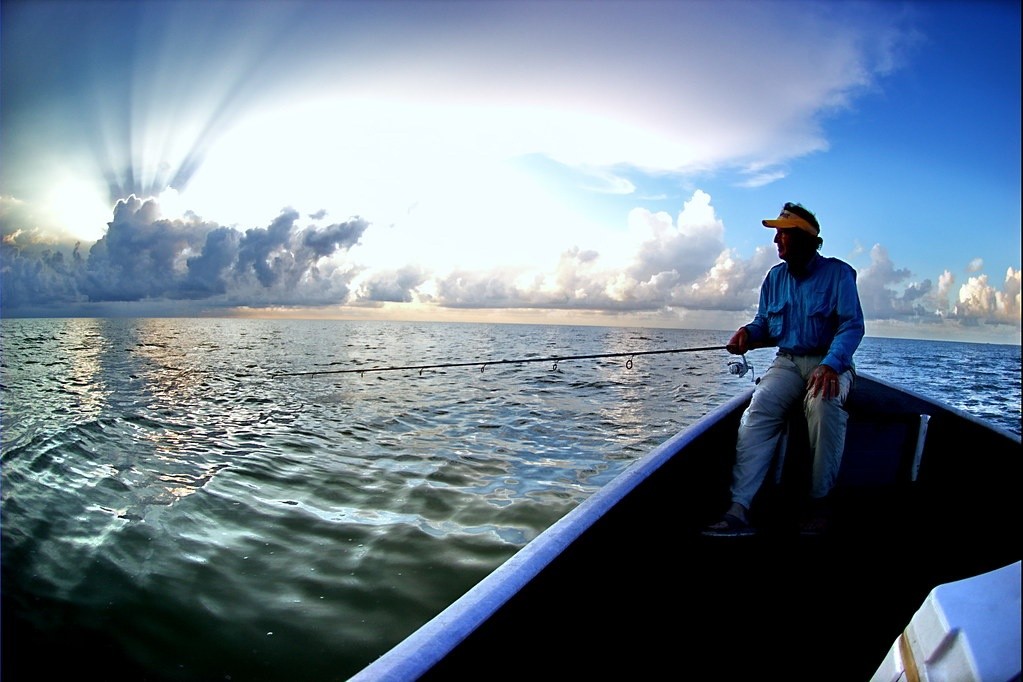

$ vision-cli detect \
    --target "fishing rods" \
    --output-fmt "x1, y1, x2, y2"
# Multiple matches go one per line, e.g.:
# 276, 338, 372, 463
266, 342, 780, 386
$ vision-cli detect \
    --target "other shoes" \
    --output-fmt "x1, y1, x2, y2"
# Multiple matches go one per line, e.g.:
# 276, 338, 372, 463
701, 514, 759, 537
799, 521, 832, 534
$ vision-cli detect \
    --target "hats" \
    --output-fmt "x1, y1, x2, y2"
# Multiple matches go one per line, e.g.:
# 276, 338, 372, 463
762, 209, 818, 236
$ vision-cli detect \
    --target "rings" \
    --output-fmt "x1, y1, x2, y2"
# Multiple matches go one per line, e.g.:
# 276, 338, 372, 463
831, 380, 835, 383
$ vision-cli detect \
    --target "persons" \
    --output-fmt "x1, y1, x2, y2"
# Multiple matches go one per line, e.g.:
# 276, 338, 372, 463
701, 204, 865, 539
